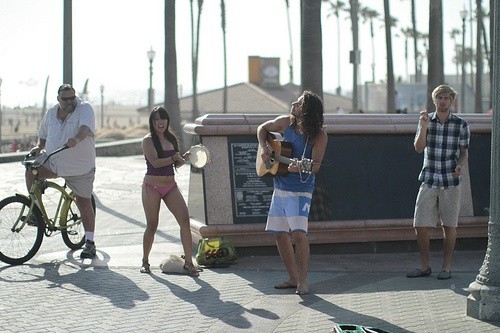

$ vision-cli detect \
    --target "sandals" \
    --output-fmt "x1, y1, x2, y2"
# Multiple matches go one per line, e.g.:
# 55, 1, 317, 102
140, 259, 151, 274
183, 260, 200, 277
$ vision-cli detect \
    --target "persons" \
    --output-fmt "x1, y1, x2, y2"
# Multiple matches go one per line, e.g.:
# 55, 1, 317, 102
406, 85, 470, 279
21, 84, 96, 258
257, 91, 327, 295
140, 107, 199, 277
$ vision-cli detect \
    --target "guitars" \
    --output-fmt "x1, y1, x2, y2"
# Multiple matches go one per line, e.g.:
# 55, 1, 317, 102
256, 131, 315, 178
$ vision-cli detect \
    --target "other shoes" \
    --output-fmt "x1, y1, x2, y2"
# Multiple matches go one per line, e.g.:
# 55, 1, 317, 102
437, 271, 452, 280
406, 267, 432, 278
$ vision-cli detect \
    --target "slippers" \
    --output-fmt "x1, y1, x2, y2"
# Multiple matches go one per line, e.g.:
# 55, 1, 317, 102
274, 282, 297, 289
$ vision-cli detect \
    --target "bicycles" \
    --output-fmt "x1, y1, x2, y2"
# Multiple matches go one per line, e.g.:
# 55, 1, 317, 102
0, 144, 96, 266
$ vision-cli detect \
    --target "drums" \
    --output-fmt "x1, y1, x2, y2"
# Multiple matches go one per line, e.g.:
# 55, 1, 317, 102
190, 145, 210, 168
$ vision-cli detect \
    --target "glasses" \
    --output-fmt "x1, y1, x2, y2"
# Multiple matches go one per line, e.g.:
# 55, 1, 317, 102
59, 96, 78, 101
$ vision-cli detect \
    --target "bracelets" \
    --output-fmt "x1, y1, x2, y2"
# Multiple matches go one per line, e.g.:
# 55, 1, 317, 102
171, 155, 174, 163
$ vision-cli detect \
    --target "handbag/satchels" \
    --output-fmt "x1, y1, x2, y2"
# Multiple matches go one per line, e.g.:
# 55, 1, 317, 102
195, 237, 241, 267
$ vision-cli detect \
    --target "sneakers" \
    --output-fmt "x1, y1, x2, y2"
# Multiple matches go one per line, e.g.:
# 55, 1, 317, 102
79, 239, 97, 259
20, 215, 38, 225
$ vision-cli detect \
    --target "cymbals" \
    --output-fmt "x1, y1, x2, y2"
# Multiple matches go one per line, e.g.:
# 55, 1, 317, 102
189, 143, 212, 169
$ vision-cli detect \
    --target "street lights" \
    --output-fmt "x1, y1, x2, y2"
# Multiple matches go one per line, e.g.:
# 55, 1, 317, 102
100, 84, 105, 129
146, 47, 155, 113
459, 4, 468, 114
